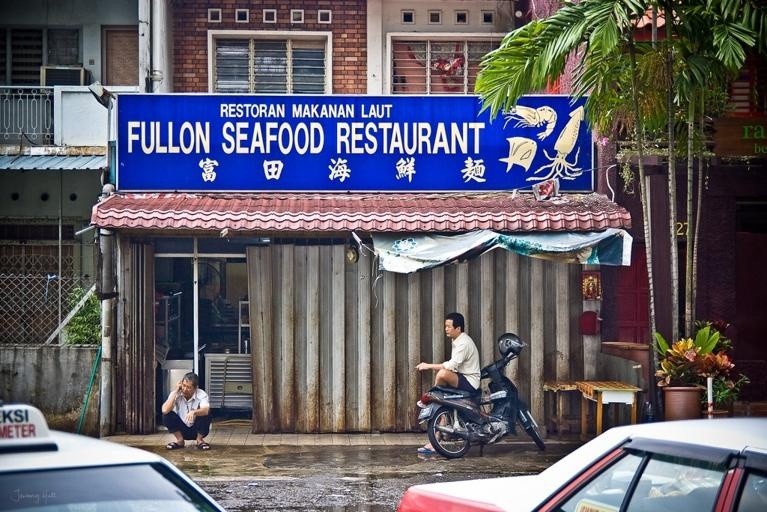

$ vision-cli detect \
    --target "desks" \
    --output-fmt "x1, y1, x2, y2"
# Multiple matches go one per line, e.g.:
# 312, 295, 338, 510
575, 381, 643, 443
541, 380, 621, 436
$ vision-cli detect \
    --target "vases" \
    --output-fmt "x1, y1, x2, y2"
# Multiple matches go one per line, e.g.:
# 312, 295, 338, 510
662, 386, 705, 421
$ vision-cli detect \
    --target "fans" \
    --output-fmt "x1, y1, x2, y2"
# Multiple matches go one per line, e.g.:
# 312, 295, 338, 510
177, 262, 222, 304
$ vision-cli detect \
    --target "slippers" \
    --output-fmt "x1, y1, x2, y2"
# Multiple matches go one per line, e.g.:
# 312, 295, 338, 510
165, 442, 184, 450
416, 447, 438, 454
196, 442, 211, 450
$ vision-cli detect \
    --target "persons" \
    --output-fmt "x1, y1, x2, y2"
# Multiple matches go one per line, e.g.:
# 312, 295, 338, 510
415, 312, 482, 452
161, 372, 213, 451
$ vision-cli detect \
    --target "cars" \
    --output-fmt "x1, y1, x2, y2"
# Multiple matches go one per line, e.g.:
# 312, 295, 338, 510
395, 416, 767, 511
0, 404, 227, 512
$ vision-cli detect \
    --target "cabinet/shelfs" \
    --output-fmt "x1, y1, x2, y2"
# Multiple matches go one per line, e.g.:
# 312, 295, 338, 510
235, 299, 250, 353
155, 290, 182, 357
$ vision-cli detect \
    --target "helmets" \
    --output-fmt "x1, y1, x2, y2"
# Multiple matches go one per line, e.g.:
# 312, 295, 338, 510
497, 333, 527, 360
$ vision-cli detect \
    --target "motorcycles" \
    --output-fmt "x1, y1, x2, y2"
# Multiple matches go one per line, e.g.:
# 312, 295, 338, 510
414, 333, 548, 459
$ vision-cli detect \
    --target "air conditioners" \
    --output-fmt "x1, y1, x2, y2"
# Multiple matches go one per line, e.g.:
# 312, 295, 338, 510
39, 63, 86, 97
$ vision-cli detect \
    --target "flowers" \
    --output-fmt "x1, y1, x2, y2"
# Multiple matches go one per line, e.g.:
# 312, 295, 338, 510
650, 324, 729, 385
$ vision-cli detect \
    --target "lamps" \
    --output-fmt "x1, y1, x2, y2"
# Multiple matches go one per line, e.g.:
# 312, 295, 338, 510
343, 234, 359, 266
87, 78, 116, 111
